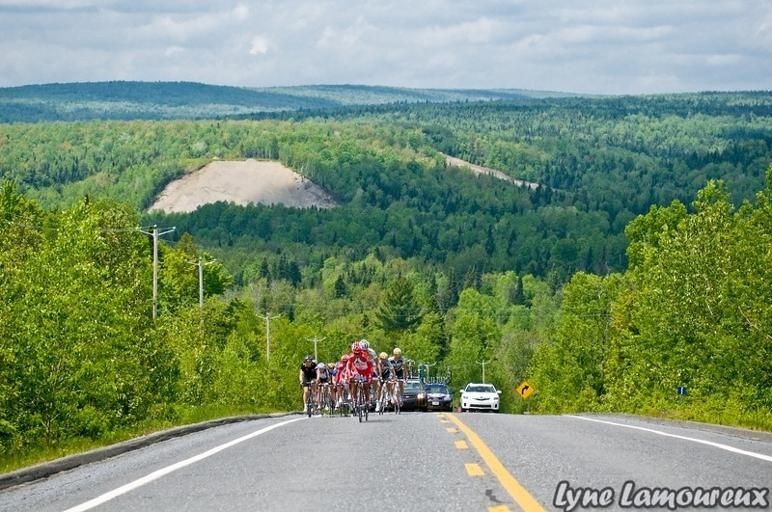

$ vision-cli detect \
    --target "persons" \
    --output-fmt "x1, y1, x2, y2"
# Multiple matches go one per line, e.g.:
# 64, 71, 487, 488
298, 338, 430, 416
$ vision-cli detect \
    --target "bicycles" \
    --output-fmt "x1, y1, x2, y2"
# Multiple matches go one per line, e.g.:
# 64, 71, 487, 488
302, 376, 407, 422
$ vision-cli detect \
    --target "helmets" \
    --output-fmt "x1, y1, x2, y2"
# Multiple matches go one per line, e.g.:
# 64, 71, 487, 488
317, 363, 327, 372
392, 347, 402, 355
351, 341, 363, 354
378, 351, 389, 360
303, 354, 315, 362
359, 339, 369, 351
341, 353, 350, 362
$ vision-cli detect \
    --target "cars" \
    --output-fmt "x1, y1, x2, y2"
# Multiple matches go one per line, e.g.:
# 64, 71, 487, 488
400, 379, 502, 413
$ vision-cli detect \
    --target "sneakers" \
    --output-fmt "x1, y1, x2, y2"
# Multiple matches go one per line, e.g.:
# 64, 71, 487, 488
302, 393, 404, 413
